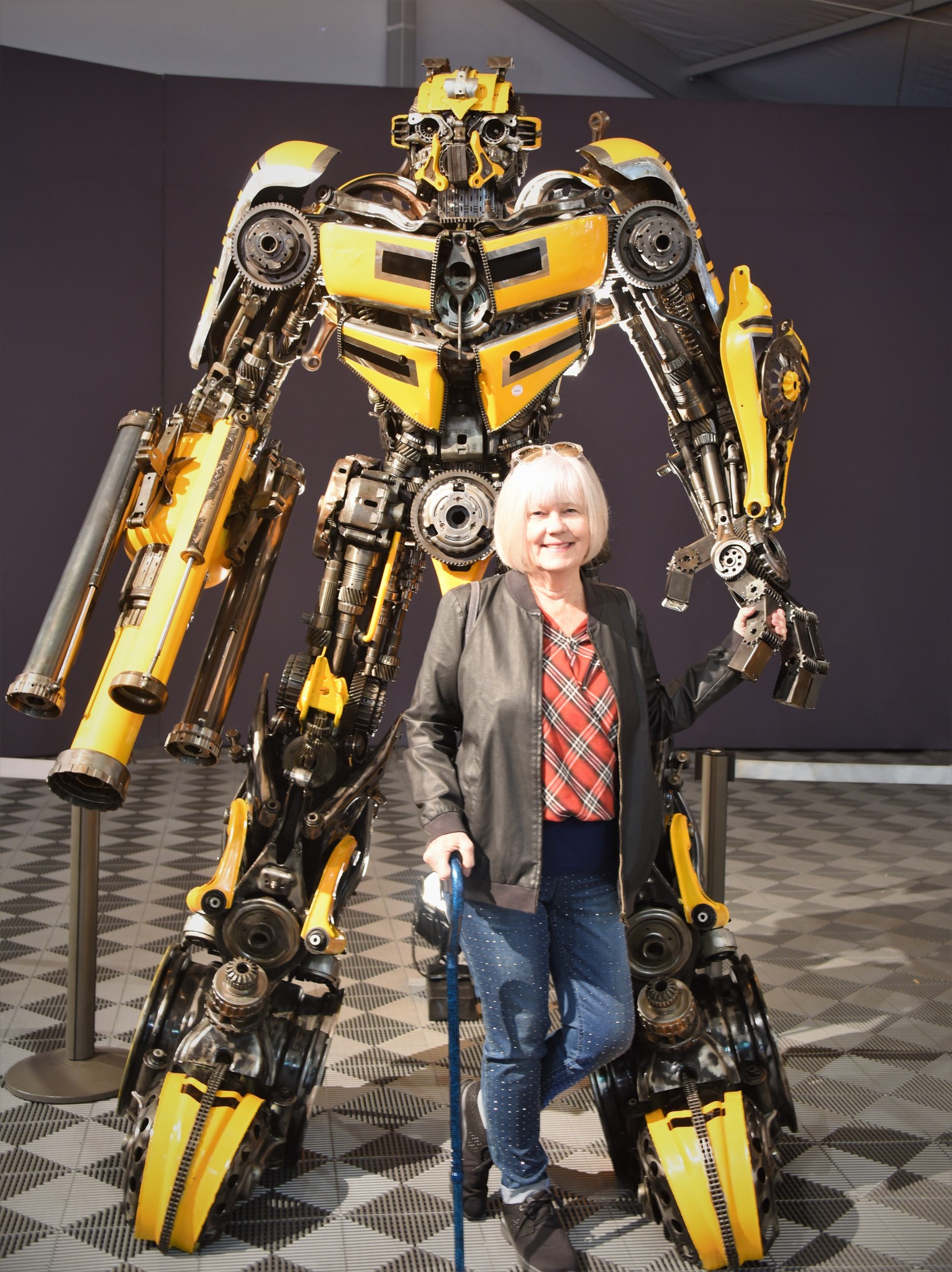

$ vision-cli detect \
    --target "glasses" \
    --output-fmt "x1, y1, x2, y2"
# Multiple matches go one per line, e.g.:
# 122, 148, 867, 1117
509, 441, 583, 472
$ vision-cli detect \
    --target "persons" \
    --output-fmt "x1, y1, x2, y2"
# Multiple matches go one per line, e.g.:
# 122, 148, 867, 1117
405, 442, 787, 1272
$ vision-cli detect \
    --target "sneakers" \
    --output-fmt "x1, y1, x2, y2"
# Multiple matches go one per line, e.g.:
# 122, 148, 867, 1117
447, 1078, 495, 1220
499, 1187, 578, 1272
